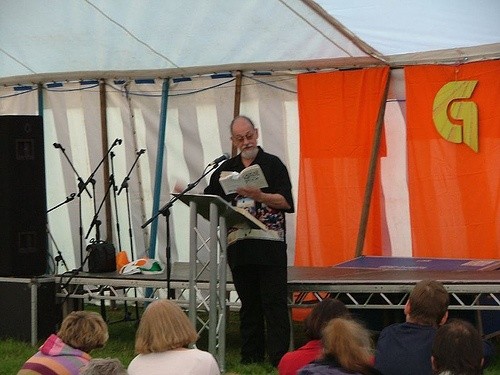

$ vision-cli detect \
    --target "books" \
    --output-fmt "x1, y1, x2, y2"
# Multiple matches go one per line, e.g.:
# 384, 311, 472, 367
219, 164, 268, 195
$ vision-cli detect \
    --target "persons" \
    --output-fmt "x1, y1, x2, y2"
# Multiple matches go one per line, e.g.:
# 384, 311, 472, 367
431, 320, 484, 375
277, 298, 351, 375
296, 318, 378, 375
17, 310, 109, 375
80, 358, 127, 375
209, 116, 295, 369
127, 300, 220, 375
374, 280, 494, 375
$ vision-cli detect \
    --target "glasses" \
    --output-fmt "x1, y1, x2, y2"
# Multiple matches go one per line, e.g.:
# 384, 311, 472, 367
235, 132, 255, 143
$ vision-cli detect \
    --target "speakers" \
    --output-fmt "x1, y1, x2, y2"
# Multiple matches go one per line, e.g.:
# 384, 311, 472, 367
0, 115, 58, 342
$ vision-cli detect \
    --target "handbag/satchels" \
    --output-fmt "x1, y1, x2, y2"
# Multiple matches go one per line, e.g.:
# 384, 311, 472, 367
86, 238, 117, 273
119, 257, 164, 275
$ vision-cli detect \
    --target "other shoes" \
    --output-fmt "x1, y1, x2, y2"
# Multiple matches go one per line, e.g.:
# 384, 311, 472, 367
271, 361, 279, 368
240, 357, 264, 365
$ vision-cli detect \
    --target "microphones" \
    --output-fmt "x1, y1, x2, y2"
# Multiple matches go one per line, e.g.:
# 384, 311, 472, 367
208, 153, 230, 166
